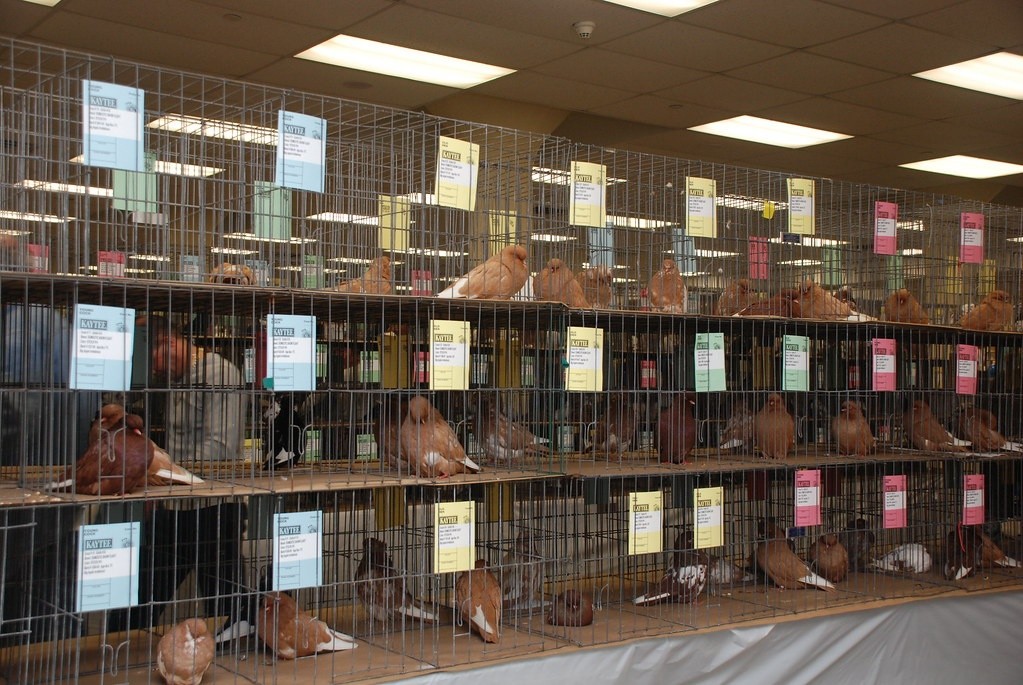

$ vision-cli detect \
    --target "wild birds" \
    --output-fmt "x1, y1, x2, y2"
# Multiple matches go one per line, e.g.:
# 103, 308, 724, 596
646, 260, 688, 312
573, 264, 613, 309
885, 289, 930, 325
796, 281, 860, 321
833, 286, 865, 313
320, 256, 393, 294
734, 288, 801, 318
961, 290, 1013, 330
532, 259, 590, 308
832, 401, 878, 460
400, 395, 481, 481
722, 394, 795, 460
581, 398, 645, 464
901, 398, 1023, 458
43, 405, 1022, 685
472, 397, 552, 468
653, 392, 696, 464
712, 279, 760, 315
207, 261, 254, 285
436, 244, 528, 301
